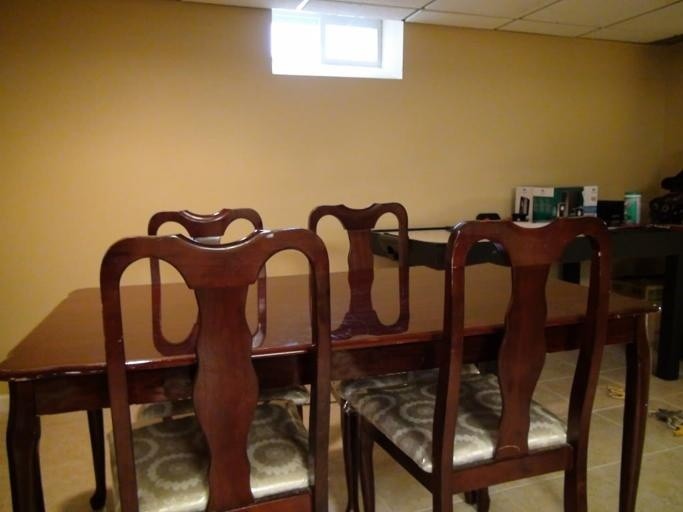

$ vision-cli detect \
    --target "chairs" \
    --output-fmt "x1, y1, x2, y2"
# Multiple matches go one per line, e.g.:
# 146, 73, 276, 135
308, 202, 476, 506
135, 207, 312, 422
99, 228, 331, 511
334, 217, 611, 512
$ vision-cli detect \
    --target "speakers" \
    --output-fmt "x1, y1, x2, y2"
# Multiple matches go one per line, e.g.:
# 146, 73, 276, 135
597, 200, 623, 227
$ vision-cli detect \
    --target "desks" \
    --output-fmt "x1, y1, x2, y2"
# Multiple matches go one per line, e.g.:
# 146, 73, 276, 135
369, 226, 682, 380
1, 259, 663, 511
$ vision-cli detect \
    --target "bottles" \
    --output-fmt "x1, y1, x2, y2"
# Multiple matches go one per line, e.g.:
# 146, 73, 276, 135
624, 191, 643, 227
